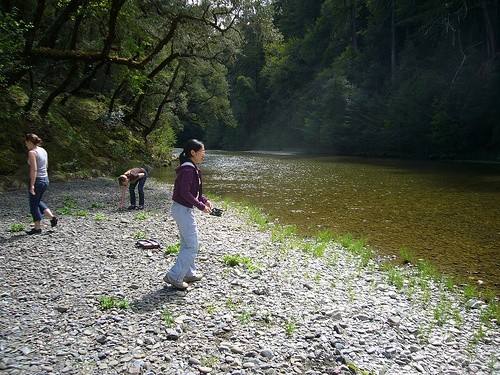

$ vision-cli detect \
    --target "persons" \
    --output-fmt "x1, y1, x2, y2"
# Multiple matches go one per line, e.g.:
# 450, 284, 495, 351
24, 133, 58, 234
118, 167, 147, 210
163, 140, 212, 289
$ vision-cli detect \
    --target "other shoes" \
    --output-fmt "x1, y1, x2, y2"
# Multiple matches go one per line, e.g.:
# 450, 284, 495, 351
138, 205, 143, 209
51, 217, 58, 227
163, 274, 188, 289
183, 273, 203, 282
128, 206, 135, 210
26, 228, 42, 235
